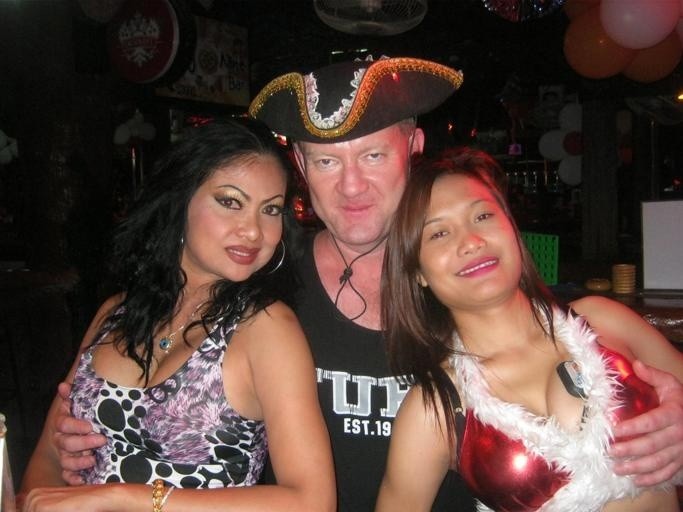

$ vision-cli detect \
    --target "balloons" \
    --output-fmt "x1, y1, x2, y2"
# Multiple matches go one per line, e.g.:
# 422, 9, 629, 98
560, 0, 683, 84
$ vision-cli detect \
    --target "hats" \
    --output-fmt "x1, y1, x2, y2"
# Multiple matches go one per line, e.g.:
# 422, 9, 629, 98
249, 49, 465, 145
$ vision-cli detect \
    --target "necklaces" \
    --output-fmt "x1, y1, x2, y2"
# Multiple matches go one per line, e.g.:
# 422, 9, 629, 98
159, 289, 219, 355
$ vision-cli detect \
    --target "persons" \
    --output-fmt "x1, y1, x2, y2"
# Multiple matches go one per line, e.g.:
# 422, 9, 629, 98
48, 53, 683, 512
16, 113, 340, 512
371, 143, 683, 511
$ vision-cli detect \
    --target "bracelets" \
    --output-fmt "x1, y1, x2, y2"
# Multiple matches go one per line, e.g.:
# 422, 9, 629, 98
159, 485, 176, 509
150, 478, 164, 512
